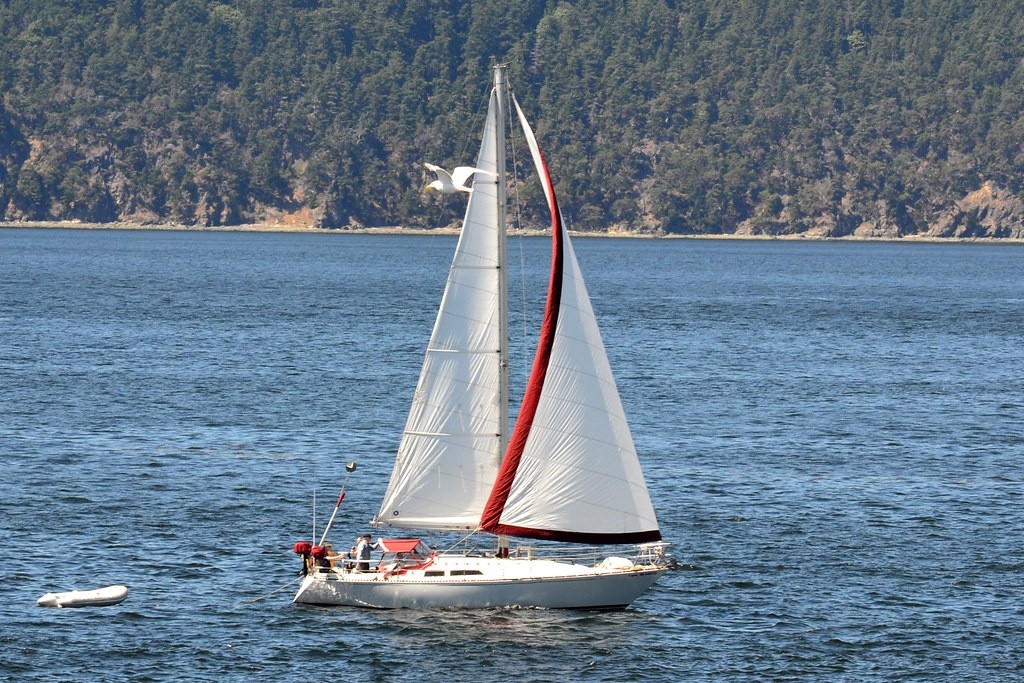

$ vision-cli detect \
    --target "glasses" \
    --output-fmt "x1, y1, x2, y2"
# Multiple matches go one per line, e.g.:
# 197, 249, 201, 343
367, 536, 371, 538
328, 546, 331, 547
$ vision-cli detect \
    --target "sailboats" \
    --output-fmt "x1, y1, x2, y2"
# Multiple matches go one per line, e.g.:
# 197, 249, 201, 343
291, 55, 680, 610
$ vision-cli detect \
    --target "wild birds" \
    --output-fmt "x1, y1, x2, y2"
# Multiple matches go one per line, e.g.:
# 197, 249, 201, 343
424, 163, 497, 194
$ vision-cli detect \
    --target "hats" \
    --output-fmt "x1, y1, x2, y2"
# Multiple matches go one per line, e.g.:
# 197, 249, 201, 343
324, 543, 332, 547
363, 533, 372, 537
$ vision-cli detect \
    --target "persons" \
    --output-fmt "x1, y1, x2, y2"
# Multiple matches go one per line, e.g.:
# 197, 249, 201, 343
323, 542, 347, 568
351, 534, 380, 573
